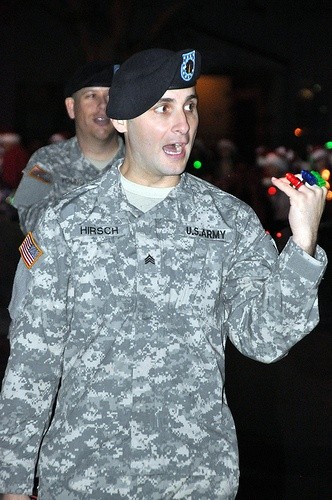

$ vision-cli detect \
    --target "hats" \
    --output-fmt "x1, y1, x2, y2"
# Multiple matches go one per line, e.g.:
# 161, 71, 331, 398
65, 60, 123, 97
106, 48, 201, 119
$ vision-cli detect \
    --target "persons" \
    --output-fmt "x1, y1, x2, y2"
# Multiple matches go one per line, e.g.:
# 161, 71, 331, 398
15, 65, 126, 235
0, 46, 329, 500
0, 130, 332, 237
